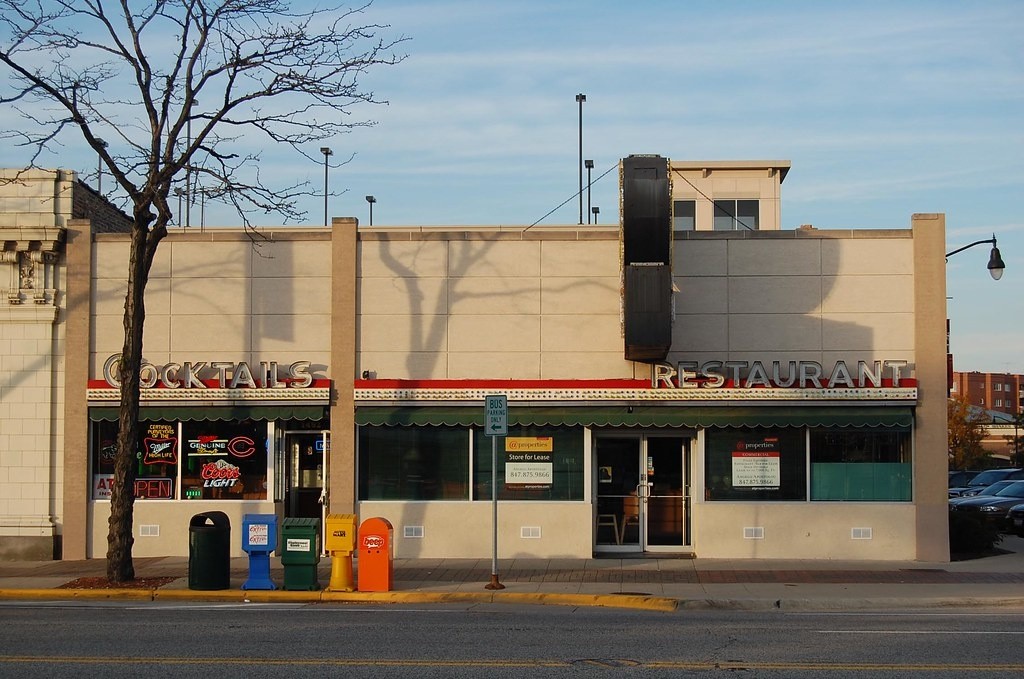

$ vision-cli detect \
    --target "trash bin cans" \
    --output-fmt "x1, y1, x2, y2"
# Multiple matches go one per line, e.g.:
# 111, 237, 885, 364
240, 514, 279, 591
325, 515, 359, 593
188, 511, 231, 590
281, 517, 322, 591
358, 516, 395, 592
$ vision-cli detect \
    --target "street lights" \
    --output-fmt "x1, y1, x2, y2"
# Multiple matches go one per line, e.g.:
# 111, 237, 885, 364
319, 146, 334, 227
186, 98, 200, 227
174, 187, 183, 227
584, 159, 595, 225
990, 414, 1019, 469
365, 195, 377, 226
575, 93, 588, 225
94, 137, 109, 195
592, 206, 600, 224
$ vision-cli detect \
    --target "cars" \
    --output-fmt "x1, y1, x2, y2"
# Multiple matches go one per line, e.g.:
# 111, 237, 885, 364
948, 467, 1024, 539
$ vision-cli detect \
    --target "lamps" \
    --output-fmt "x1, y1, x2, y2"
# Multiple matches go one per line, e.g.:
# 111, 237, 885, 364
946, 234, 1006, 279
627, 407, 634, 413
363, 370, 370, 380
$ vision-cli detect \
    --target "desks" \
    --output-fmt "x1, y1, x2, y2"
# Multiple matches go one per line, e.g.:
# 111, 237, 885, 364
174, 479, 222, 498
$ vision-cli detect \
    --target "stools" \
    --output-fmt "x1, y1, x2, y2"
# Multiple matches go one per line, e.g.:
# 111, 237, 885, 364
597, 514, 619, 545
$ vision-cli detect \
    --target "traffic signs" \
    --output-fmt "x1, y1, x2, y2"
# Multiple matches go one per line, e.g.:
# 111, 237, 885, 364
485, 393, 509, 437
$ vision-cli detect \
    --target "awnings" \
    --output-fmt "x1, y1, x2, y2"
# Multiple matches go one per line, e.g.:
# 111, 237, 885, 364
353, 407, 914, 427
89, 407, 329, 421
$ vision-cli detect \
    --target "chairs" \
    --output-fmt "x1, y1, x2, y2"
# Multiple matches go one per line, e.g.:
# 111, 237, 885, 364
621, 499, 639, 543
